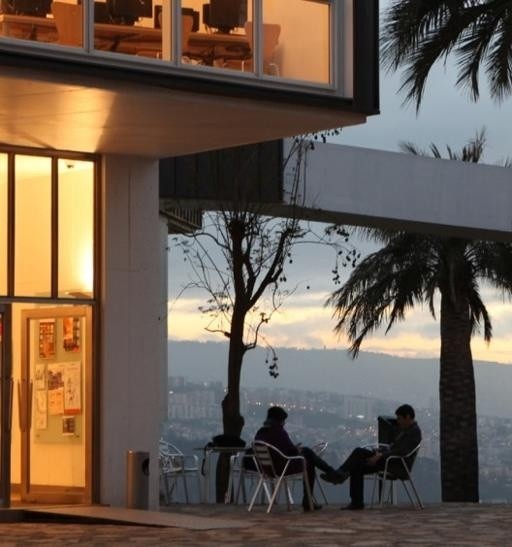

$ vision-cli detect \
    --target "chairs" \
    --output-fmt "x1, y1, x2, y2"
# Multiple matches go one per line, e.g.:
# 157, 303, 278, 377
0, 0, 281, 76
158, 437, 423, 514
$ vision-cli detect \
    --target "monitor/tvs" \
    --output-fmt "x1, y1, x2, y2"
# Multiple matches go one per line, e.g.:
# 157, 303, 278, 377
0, 0, 53, 19
94, 0, 247, 35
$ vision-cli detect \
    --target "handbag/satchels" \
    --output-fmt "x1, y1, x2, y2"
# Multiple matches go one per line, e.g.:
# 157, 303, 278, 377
201, 434, 248, 475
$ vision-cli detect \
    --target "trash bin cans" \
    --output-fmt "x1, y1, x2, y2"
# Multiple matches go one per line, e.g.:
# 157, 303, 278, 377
127, 450, 150, 510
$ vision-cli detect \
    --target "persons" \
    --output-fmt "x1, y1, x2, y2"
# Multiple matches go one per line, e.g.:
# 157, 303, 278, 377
255, 404, 336, 511
319, 402, 420, 510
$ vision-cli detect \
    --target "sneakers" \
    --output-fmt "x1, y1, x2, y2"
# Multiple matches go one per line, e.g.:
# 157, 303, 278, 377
320, 465, 346, 484
340, 500, 364, 511
302, 498, 322, 512
